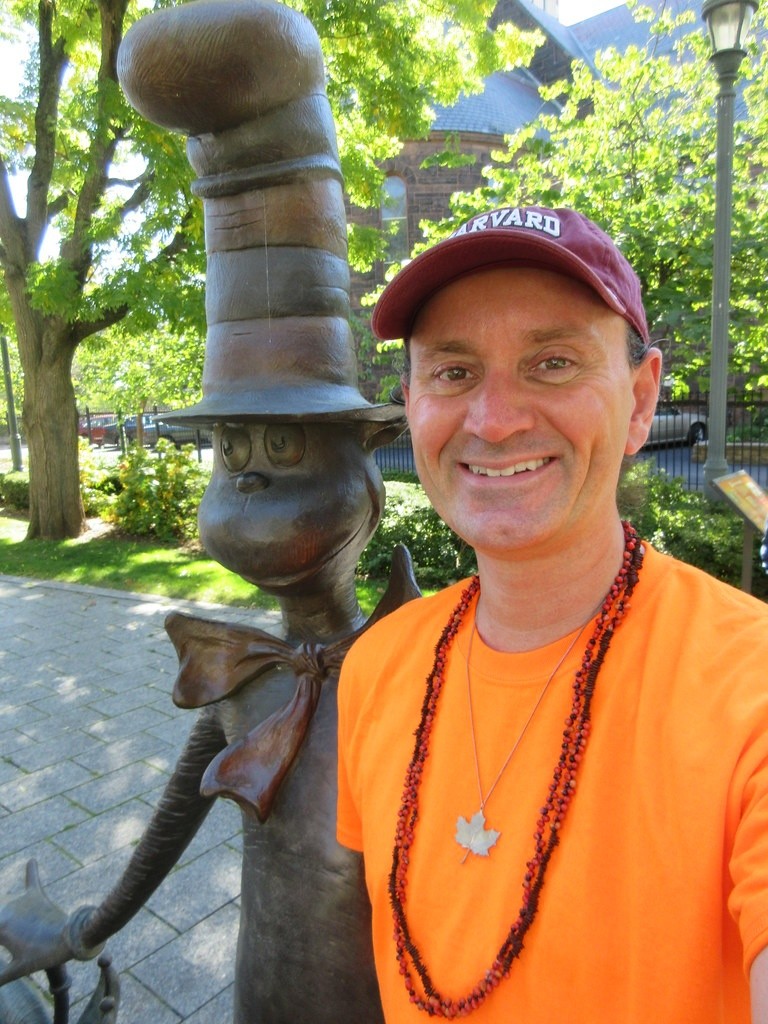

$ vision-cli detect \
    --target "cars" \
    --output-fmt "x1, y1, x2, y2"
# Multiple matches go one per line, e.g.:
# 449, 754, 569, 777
639, 397, 708, 452
78, 412, 215, 451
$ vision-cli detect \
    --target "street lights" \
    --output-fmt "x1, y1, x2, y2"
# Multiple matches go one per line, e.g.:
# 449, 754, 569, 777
683, 0, 761, 505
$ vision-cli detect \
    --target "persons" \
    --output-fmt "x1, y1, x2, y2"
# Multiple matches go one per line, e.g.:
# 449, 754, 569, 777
335, 205, 768, 1024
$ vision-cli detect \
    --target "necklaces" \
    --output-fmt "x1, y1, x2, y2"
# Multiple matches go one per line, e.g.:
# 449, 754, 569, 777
457, 593, 606, 863
386, 522, 645, 1020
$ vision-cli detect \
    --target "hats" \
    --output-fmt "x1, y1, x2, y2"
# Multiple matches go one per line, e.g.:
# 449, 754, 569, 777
371, 206, 650, 359
